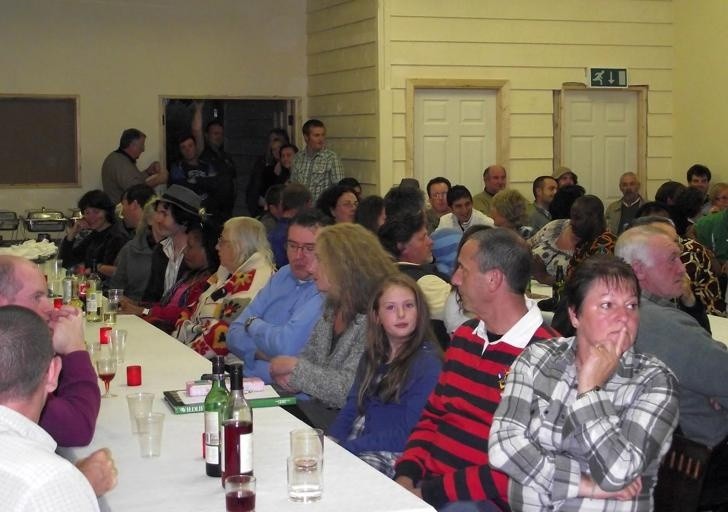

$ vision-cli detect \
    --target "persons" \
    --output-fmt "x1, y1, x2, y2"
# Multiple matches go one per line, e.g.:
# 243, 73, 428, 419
0, 253, 102, 446
103, 128, 167, 205
167, 99, 345, 208
62, 164, 727, 512
0, 303, 118, 511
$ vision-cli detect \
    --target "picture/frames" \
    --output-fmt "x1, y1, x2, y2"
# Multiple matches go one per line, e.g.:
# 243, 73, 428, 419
1, 93, 81, 190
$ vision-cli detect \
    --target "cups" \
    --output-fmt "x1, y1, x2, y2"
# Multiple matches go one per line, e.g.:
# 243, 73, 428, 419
224, 474, 257, 511
127, 392, 155, 434
135, 411, 166, 459
286, 455, 321, 501
288, 426, 324, 474
39, 257, 142, 400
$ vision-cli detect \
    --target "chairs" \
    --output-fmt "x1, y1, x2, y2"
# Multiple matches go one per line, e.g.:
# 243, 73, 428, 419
653, 436, 711, 512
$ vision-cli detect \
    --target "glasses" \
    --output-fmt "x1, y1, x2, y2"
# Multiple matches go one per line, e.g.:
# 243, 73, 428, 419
283, 239, 315, 254
217, 238, 232, 247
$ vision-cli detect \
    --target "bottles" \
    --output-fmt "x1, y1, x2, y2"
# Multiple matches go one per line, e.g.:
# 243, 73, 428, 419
523, 274, 531, 294
220, 367, 255, 488
203, 358, 232, 478
552, 264, 568, 306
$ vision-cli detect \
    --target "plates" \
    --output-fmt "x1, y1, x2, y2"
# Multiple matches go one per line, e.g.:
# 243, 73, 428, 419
525, 292, 551, 302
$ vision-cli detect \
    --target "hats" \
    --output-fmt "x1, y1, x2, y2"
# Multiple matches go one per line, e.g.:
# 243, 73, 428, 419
157, 184, 204, 218
553, 166, 577, 185
